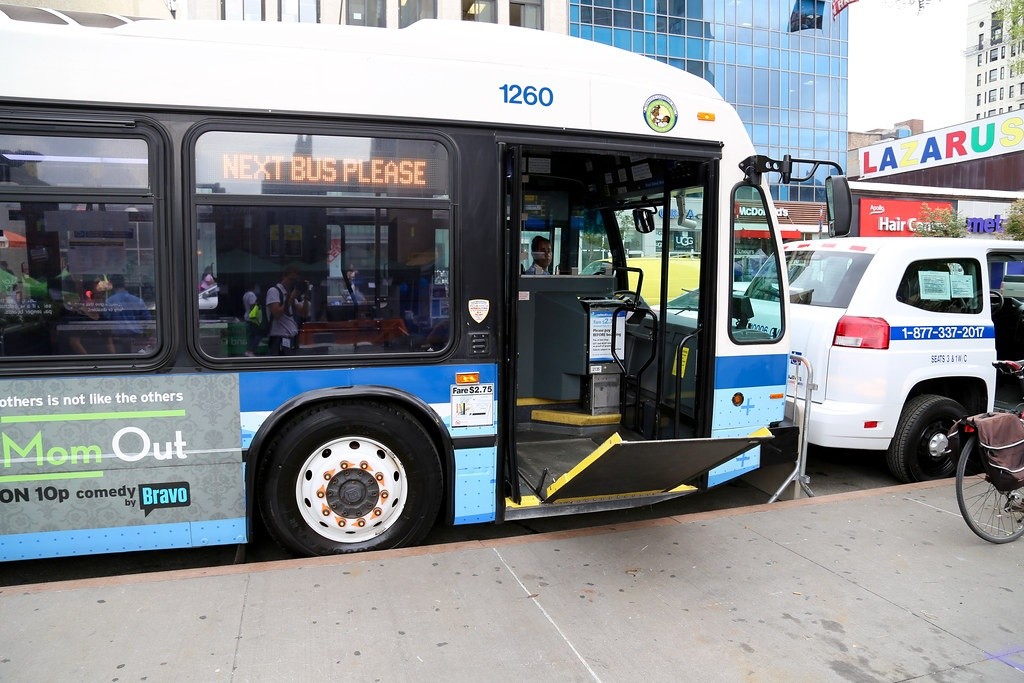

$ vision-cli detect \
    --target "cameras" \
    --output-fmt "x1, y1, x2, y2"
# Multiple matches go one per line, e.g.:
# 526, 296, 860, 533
293, 282, 308, 294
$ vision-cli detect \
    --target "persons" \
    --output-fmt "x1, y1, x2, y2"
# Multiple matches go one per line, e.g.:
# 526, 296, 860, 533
266, 266, 311, 356
526, 235, 552, 275
338, 263, 366, 305
70, 274, 152, 355
243, 282, 263, 357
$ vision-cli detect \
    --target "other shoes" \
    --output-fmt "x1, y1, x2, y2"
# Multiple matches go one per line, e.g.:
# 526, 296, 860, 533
244, 351, 255, 357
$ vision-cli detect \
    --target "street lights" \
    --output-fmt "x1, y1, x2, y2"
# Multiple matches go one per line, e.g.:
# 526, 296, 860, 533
124, 208, 142, 300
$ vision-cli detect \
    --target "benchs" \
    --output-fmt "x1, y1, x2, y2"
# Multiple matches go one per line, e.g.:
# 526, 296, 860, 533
297, 319, 410, 355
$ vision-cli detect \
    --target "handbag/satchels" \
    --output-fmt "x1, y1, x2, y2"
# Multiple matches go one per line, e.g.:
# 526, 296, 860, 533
947, 411, 1024, 491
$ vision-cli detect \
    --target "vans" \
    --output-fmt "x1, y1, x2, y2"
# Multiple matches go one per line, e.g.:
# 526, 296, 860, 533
578, 250, 703, 307
737, 240, 1024, 486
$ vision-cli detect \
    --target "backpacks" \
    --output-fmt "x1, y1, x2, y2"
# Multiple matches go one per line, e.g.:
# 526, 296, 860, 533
247, 284, 283, 338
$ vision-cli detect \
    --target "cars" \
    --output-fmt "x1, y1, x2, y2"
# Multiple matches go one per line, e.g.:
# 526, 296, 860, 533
649, 280, 806, 320
198, 282, 219, 310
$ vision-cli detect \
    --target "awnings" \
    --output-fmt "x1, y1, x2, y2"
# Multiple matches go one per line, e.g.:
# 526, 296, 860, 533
0, 229, 27, 248
734, 215, 801, 239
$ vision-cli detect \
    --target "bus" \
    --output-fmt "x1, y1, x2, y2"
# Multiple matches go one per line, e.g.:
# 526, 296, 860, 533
0, 17, 855, 566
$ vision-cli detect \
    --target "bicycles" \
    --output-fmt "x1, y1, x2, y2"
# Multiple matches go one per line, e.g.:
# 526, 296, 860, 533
954, 359, 1024, 544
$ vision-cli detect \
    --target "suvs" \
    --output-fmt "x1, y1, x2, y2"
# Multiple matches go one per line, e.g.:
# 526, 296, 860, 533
1000, 274, 1024, 298
